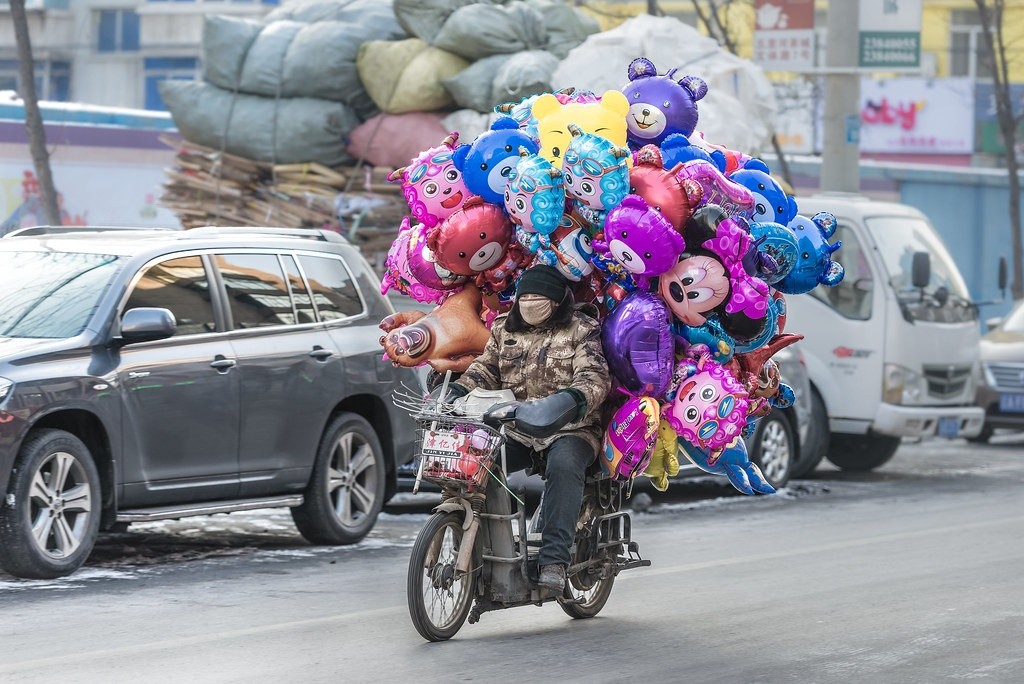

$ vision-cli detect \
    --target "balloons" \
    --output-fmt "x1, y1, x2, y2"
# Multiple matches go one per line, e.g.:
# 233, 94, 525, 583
381, 56, 843, 494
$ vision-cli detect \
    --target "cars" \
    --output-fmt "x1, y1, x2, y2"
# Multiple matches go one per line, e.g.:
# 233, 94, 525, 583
971, 292, 1024, 444
416, 339, 812, 495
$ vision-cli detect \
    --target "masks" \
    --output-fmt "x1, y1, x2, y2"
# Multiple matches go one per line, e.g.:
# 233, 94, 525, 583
519, 297, 553, 325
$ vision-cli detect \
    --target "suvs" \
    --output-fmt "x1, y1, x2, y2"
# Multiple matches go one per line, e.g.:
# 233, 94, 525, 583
0, 221, 431, 578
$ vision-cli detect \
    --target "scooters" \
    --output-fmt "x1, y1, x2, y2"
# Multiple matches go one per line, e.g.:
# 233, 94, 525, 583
407, 386, 650, 643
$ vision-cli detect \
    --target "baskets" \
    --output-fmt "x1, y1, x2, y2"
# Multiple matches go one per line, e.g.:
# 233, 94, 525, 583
413, 410, 508, 494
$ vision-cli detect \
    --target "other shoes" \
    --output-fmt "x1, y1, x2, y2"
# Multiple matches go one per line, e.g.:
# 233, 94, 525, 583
538, 564, 565, 591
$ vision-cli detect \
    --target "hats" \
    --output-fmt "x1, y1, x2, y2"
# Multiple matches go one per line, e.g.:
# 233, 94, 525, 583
517, 265, 566, 303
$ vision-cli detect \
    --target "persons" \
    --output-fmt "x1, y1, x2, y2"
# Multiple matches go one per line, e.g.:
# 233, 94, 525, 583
456, 264, 612, 590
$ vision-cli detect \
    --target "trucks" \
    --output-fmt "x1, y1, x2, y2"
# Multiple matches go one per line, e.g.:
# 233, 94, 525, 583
782, 183, 992, 474
